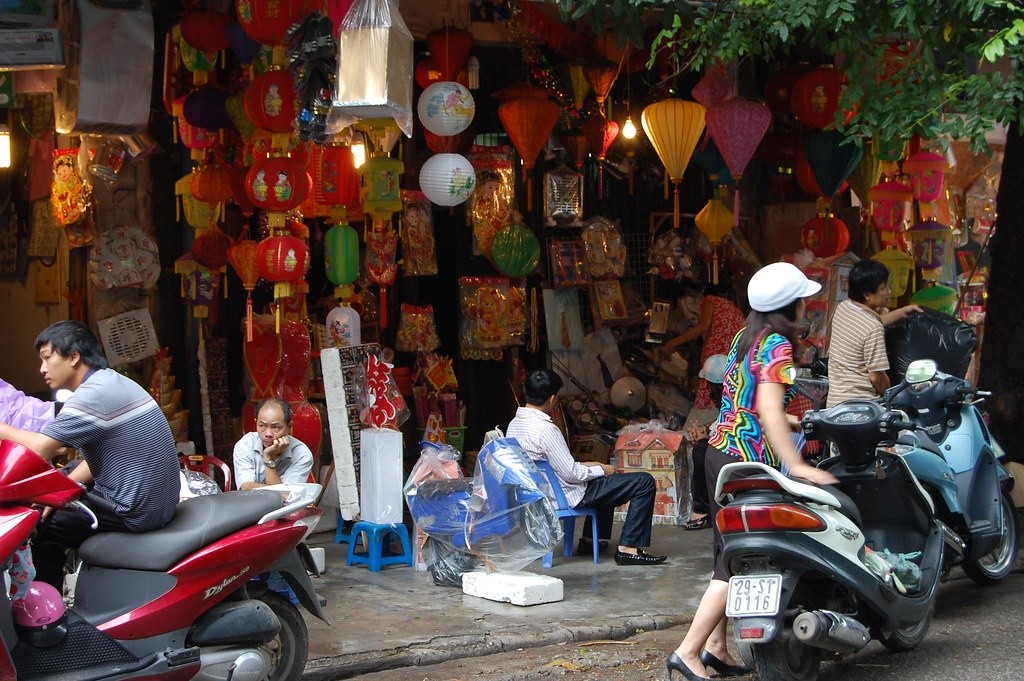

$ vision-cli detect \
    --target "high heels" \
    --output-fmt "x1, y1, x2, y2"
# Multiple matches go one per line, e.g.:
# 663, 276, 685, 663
665, 652, 716, 681
700, 651, 750, 677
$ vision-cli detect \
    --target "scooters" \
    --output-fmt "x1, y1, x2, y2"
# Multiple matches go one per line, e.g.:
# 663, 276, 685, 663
830, 369, 1021, 588
0, 440, 326, 681
714, 358, 943, 681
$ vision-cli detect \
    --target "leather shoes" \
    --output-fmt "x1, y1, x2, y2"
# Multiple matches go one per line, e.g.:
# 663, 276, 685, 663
614, 548, 667, 565
577, 538, 609, 555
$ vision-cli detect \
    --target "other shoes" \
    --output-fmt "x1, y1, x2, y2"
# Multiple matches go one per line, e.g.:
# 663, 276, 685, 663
683, 514, 713, 529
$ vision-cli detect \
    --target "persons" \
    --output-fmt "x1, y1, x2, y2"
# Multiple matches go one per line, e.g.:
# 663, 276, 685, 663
660, 282, 745, 440
233, 398, 314, 502
682, 353, 729, 530
667, 263, 840, 680
827, 260, 924, 409
0, 319, 182, 602
506, 369, 668, 566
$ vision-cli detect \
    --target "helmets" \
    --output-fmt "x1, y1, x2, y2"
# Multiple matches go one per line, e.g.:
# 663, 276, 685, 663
11, 581, 69, 647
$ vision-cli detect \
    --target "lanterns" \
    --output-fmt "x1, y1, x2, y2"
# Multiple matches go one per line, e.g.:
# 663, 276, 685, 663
172, 1, 1000, 346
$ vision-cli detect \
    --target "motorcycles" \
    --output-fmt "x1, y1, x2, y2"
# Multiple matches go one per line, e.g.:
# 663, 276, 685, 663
415, 433, 560, 556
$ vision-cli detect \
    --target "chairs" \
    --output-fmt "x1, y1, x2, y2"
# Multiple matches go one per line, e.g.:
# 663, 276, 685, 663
513, 459, 600, 570
179, 454, 232, 493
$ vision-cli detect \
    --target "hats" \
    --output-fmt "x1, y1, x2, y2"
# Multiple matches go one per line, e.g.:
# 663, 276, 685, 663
699, 353, 730, 383
747, 261, 823, 312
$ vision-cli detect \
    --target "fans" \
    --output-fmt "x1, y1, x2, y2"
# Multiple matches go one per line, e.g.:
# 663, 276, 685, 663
97, 307, 160, 368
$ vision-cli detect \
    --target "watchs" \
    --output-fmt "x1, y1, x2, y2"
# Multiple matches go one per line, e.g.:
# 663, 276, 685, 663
264, 460, 276, 468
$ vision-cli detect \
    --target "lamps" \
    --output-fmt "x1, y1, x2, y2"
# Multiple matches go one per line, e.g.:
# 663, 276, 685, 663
0, 124, 11, 169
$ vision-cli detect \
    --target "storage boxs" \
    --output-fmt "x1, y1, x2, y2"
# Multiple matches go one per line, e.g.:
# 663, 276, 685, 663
417, 425, 468, 468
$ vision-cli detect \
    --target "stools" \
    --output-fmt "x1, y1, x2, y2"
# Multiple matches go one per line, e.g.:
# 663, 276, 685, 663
334, 510, 414, 573
